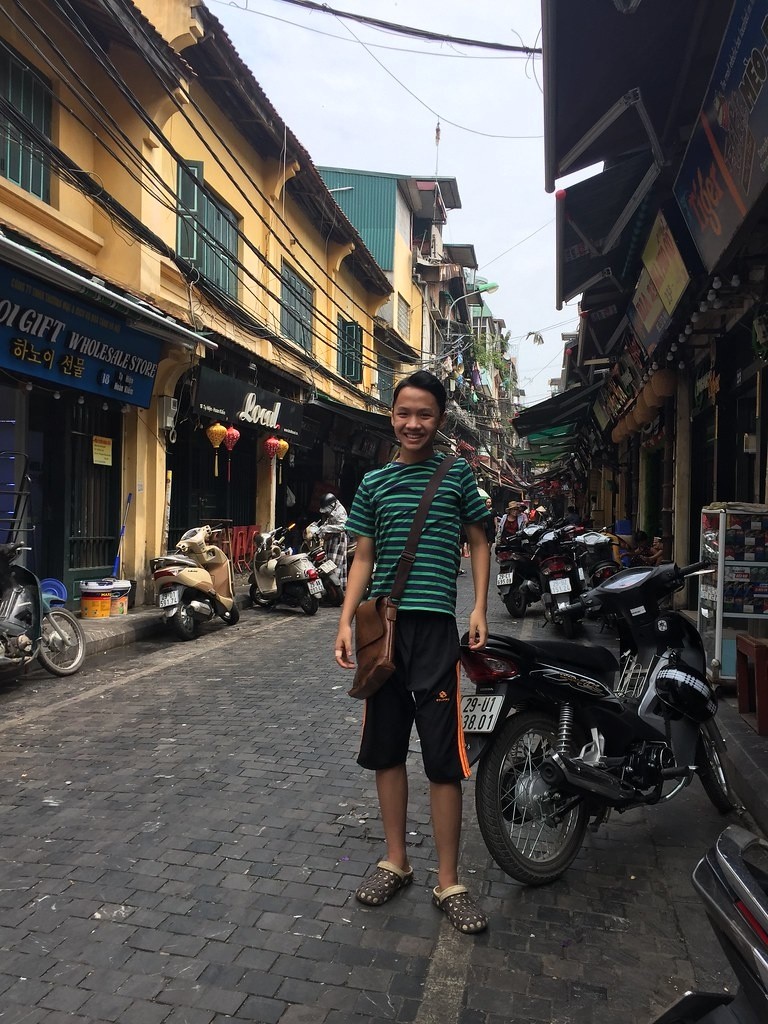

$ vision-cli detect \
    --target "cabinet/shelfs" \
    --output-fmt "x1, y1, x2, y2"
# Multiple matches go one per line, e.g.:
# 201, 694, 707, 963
697, 507, 768, 695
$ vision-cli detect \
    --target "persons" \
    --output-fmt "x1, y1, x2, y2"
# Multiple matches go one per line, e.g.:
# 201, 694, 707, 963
335, 370, 491, 935
564, 506, 579, 523
619, 530, 663, 567
483, 498, 528, 556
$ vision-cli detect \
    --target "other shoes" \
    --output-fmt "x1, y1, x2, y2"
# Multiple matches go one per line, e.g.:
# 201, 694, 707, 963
458, 569, 467, 575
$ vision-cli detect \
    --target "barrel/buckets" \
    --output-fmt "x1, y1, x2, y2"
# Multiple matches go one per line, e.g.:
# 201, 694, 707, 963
79, 580, 113, 619
102, 579, 132, 616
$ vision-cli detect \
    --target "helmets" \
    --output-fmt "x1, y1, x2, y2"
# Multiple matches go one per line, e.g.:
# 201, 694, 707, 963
320, 493, 336, 508
656, 662, 718, 722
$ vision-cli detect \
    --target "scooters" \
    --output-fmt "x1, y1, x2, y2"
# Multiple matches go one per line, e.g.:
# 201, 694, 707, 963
0, 541, 87, 677
248, 523, 327, 614
147, 522, 239, 640
298, 518, 345, 608
492, 509, 627, 638
645, 820, 768, 1024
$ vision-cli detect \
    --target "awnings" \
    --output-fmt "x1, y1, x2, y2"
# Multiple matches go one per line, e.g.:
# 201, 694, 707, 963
541, 0, 734, 311
511, 384, 596, 467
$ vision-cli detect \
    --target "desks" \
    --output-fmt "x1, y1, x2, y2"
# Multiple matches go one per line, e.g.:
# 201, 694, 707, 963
216, 523, 261, 573
735, 633, 768, 735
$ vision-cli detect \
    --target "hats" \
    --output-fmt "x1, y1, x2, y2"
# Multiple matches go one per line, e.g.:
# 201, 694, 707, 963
536, 506, 546, 512
617, 534, 634, 548
505, 501, 520, 513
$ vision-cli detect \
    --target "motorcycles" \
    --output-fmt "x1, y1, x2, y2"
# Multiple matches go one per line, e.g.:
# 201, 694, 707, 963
460, 560, 734, 888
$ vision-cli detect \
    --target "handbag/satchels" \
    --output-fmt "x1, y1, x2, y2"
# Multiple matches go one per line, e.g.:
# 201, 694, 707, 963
348, 595, 397, 700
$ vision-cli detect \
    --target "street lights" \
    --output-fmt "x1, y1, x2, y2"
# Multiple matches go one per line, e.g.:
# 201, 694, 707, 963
448, 281, 501, 344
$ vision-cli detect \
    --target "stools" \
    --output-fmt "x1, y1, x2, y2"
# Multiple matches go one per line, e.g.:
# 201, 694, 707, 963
615, 519, 632, 566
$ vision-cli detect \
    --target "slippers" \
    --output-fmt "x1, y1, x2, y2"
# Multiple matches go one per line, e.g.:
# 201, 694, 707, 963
356, 860, 414, 906
431, 885, 490, 934
